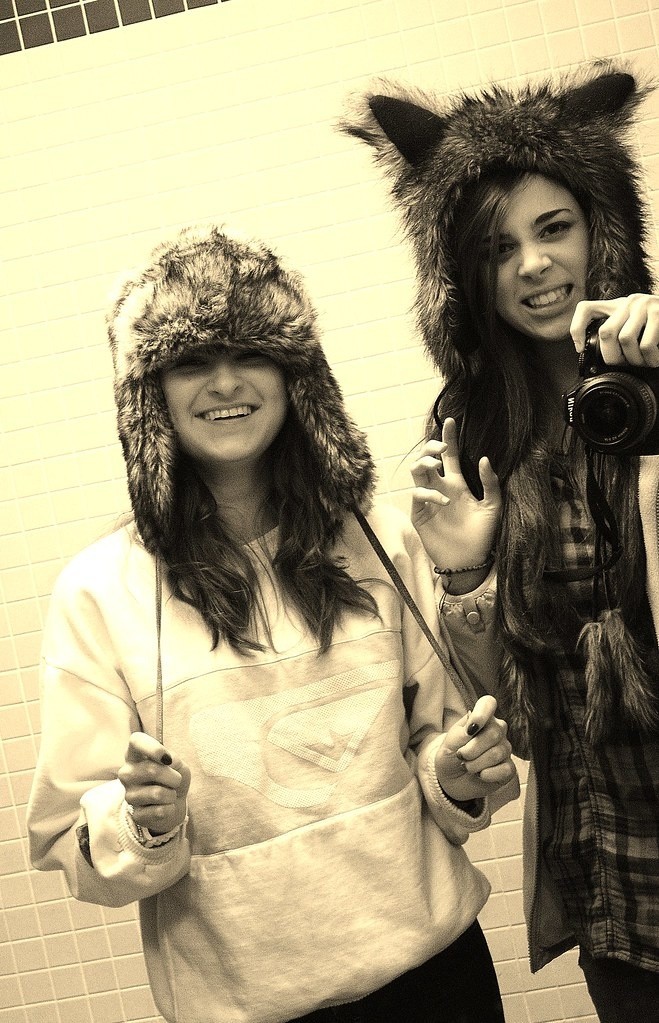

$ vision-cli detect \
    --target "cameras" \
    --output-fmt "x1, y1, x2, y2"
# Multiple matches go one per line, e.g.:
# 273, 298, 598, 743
561, 316, 659, 456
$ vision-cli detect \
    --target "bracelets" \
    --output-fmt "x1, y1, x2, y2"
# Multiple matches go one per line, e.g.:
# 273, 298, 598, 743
433, 560, 490, 610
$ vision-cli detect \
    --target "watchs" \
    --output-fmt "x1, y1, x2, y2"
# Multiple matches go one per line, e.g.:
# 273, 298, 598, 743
126, 807, 181, 849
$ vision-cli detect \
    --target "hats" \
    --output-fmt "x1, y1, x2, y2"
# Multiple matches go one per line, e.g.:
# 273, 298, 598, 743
368, 70, 654, 760
107, 231, 372, 551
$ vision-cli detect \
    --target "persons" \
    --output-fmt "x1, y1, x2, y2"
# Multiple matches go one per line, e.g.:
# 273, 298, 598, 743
25, 226, 521, 1023
342, 70, 659, 1023
